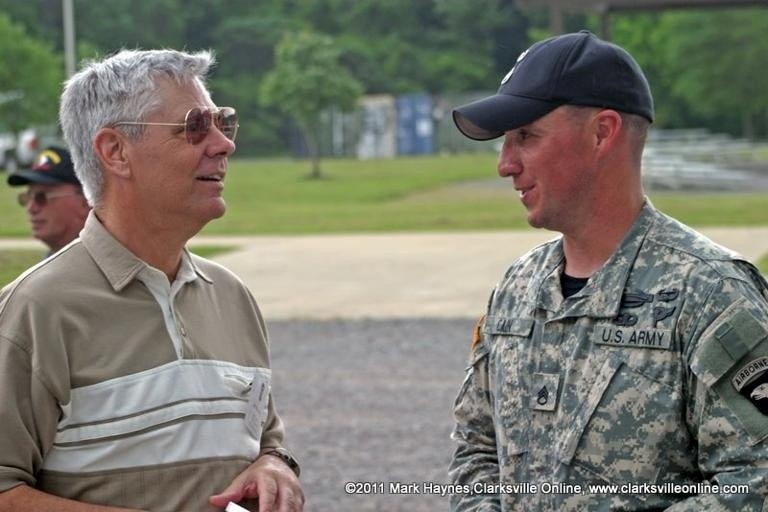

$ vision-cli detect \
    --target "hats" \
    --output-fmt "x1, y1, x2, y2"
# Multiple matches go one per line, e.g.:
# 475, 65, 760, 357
8, 146, 82, 185
453, 30, 655, 141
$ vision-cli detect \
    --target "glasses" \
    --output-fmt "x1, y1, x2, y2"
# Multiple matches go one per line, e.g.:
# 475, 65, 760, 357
17, 193, 76, 206
113, 106, 240, 144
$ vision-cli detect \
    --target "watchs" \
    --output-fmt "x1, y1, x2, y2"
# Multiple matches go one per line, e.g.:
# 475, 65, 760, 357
260, 447, 301, 477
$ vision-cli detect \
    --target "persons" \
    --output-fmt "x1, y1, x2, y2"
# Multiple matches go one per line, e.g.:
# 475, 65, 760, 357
1, 46, 305, 512
8, 144, 93, 255
449, 29, 768, 512
